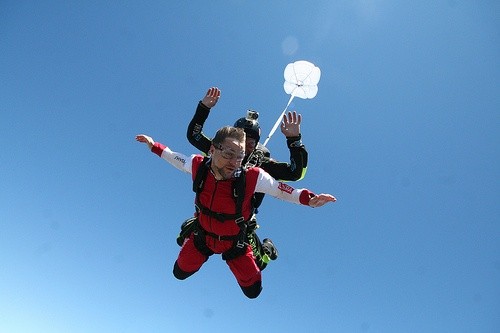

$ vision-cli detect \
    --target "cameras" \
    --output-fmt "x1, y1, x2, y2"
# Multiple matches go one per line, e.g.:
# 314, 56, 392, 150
247, 110, 259, 121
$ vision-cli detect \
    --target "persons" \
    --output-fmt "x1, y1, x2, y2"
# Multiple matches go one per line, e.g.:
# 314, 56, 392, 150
176, 88, 309, 270
135, 126, 337, 299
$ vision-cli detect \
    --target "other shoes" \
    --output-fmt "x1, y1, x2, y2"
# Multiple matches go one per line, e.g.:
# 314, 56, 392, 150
262, 238, 278, 260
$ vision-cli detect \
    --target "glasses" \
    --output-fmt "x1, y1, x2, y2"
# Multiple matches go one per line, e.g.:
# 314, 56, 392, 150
216, 144, 245, 162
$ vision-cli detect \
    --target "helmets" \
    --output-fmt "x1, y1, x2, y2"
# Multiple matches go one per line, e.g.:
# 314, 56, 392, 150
233, 110, 261, 148
